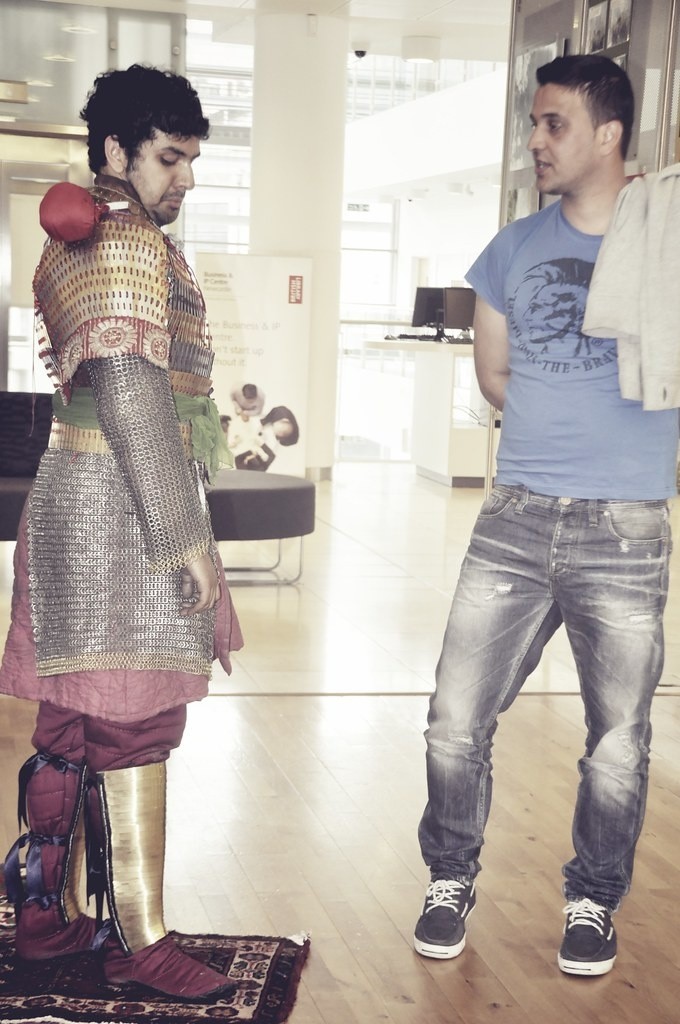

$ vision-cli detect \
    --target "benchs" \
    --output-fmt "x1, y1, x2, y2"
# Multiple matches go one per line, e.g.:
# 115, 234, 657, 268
0, 391, 54, 543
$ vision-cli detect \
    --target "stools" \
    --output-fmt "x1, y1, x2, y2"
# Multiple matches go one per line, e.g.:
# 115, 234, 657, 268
204, 468, 315, 586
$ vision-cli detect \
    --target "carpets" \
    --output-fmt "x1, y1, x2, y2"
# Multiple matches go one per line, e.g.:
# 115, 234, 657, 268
0, 928, 311, 1024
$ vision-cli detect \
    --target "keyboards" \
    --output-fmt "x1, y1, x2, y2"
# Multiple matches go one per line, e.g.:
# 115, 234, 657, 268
440, 336, 474, 344
397, 334, 434, 340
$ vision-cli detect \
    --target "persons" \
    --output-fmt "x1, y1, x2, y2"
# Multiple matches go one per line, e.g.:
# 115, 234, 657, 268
414, 54, 680, 976
219, 384, 293, 464
0, 63, 240, 1003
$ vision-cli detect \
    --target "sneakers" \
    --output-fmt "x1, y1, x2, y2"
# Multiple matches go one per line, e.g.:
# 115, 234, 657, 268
557, 898, 618, 975
413, 879, 478, 959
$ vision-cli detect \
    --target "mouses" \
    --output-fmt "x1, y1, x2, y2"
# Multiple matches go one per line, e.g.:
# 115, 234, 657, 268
384, 335, 396, 340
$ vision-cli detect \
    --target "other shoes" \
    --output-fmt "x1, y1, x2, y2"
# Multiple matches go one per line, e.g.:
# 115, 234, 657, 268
106, 929, 239, 1000
16, 912, 122, 960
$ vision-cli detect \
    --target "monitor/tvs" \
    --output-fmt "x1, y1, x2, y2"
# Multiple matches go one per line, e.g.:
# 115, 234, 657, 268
412, 288, 476, 341
